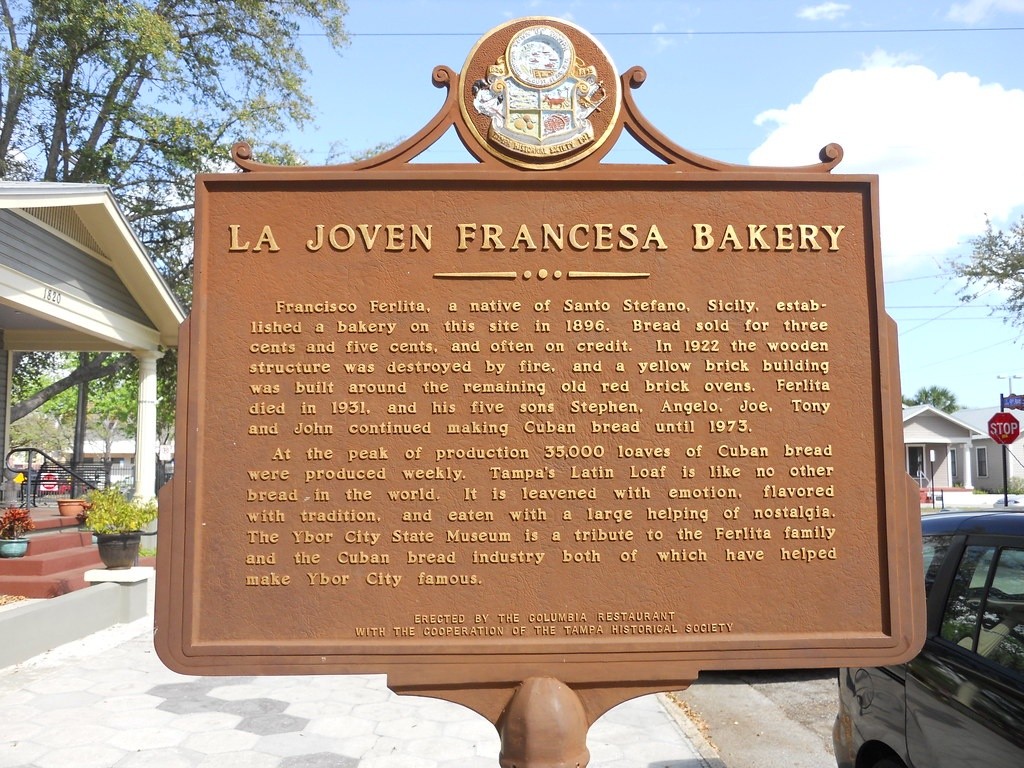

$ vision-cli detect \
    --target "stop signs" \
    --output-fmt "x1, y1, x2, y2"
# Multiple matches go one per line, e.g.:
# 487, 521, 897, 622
985, 409, 1021, 445
40, 473, 59, 492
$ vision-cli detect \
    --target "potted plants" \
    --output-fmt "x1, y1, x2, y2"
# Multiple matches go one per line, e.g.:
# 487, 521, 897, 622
75, 510, 94, 547
84, 481, 158, 569
0, 504, 36, 557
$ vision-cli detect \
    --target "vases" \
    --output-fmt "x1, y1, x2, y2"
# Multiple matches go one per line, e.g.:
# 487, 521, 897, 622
56, 499, 85, 516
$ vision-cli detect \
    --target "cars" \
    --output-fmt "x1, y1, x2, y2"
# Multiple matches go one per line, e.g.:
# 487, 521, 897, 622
827, 501, 1023, 768
